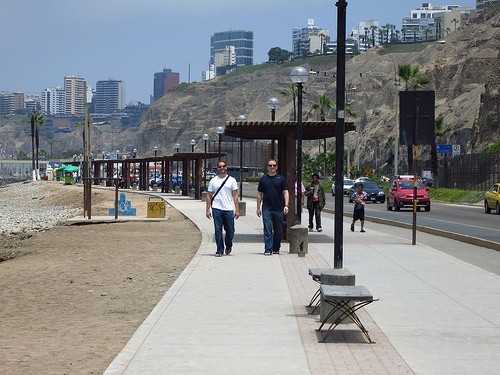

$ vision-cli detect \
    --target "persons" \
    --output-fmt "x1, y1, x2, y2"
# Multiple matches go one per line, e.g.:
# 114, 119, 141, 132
295, 170, 306, 213
73, 172, 77, 183
351, 183, 366, 232
305, 173, 325, 232
206, 161, 239, 257
257, 158, 289, 255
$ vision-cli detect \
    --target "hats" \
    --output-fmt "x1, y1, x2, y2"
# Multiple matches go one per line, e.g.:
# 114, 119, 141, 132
311, 174, 319, 178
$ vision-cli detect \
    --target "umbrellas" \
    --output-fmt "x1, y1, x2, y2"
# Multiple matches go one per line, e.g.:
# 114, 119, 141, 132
57, 166, 79, 173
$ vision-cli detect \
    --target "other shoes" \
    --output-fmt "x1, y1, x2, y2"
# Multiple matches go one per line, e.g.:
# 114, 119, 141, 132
317, 227, 322, 232
309, 228, 313, 232
264, 250, 272, 256
360, 229, 365, 232
273, 249, 279, 254
215, 252, 224, 257
351, 224, 354, 231
225, 245, 233, 255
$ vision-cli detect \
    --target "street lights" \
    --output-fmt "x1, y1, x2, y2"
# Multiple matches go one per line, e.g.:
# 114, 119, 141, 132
69, 144, 182, 195
202, 134, 210, 189
291, 64, 310, 226
236, 113, 246, 204
190, 138, 196, 189
267, 96, 281, 166
216, 126, 225, 161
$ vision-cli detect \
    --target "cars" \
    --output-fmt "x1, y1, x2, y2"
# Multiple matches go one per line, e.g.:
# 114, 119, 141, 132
330, 179, 354, 195
387, 178, 430, 211
91, 166, 217, 190
349, 181, 386, 204
483, 182, 500, 215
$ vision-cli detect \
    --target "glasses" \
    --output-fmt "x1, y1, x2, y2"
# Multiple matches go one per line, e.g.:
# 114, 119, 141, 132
268, 165, 277, 167
217, 165, 226, 168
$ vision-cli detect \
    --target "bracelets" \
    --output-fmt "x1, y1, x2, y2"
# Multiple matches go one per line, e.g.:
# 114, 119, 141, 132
285, 205, 289, 207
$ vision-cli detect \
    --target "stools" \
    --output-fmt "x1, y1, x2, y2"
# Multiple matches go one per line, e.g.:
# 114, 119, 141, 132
314, 285, 376, 344
306, 268, 332, 314
